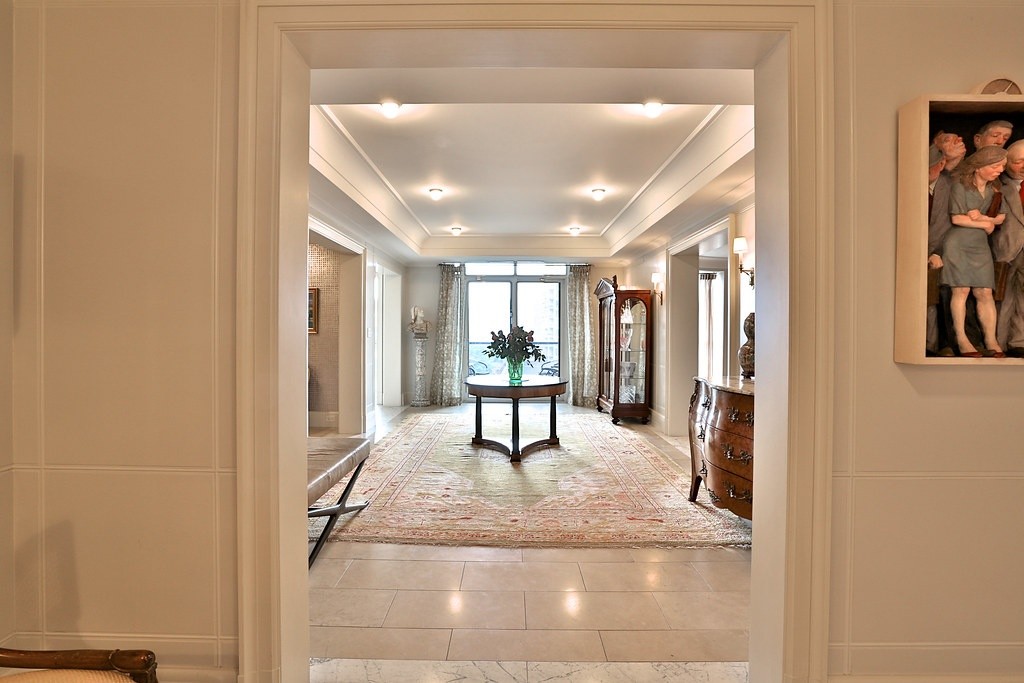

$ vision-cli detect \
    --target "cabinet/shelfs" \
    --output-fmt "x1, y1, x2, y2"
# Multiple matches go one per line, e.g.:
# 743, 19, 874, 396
688, 374, 754, 523
595, 288, 652, 424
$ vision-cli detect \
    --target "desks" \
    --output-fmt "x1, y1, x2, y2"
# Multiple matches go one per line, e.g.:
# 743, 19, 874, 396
465, 373, 569, 463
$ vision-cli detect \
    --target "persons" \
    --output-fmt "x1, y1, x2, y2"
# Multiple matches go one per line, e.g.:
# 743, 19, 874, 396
406, 306, 434, 338
925, 120, 1024, 357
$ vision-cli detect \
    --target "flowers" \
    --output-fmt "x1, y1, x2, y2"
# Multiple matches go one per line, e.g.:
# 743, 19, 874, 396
484, 326, 545, 362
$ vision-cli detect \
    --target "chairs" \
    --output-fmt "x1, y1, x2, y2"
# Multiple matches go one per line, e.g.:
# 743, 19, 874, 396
0, 645, 158, 683
468, 361, 489, 375
539, 360, 559, 375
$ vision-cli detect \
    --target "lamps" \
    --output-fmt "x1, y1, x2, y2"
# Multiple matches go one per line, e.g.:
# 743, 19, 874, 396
642, 102, 663, 118
592, 188, 606, 201
733, 236, 754, 292
452, 227, 461, 235
570, 227, 580, 236
429, 188, 443, 200
381, 102, 402, 119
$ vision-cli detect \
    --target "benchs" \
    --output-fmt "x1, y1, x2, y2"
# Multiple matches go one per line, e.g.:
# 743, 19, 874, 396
308, 435, 373, 568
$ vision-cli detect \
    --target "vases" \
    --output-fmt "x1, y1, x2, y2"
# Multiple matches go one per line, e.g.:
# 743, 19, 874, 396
506, 355, 525, 383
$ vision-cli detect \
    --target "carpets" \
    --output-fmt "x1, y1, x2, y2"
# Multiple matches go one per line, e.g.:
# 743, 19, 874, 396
307, 411, 752, 550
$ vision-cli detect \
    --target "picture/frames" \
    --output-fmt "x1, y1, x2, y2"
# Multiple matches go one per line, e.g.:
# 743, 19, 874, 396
308, 285, 319, 333
